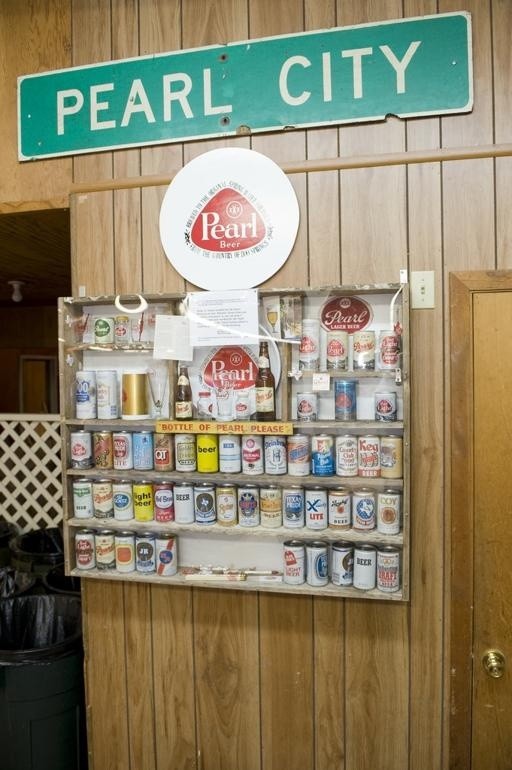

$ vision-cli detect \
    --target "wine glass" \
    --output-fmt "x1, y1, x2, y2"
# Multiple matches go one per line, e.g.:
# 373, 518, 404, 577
267, 310, 280, 336
146, 367, 168, 420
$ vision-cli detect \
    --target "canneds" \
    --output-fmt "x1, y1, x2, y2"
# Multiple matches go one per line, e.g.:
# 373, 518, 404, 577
298, 319, 401, 372
76, 370, 121, 420
296, 380, 398, 423
94, 316, 130, 344
70, 430, 404, 479
75, 529, 179, 577
282, 539, 401, 592
73, 479, 402, 534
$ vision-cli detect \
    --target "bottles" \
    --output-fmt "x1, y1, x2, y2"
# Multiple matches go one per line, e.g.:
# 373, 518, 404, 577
197, 391, 251, 423
174, 364, 193, 419
114, 315, 131, 342
253, 341, 276, 421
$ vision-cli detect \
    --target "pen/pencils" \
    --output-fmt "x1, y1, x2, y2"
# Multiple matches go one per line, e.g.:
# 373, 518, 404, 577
210, 569, 277, 574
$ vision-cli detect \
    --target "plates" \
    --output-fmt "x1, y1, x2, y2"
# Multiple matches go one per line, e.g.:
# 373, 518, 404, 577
181, 324, 282, 418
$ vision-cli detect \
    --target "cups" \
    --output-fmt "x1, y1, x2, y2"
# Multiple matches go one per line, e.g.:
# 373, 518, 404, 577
212, 371, 235, 417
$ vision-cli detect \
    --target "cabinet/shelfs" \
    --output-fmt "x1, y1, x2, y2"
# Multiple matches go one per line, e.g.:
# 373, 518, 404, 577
56, 282, 415, 603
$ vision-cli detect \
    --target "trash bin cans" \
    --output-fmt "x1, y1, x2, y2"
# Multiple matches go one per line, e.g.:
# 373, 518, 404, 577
0, 522, 88, 770
